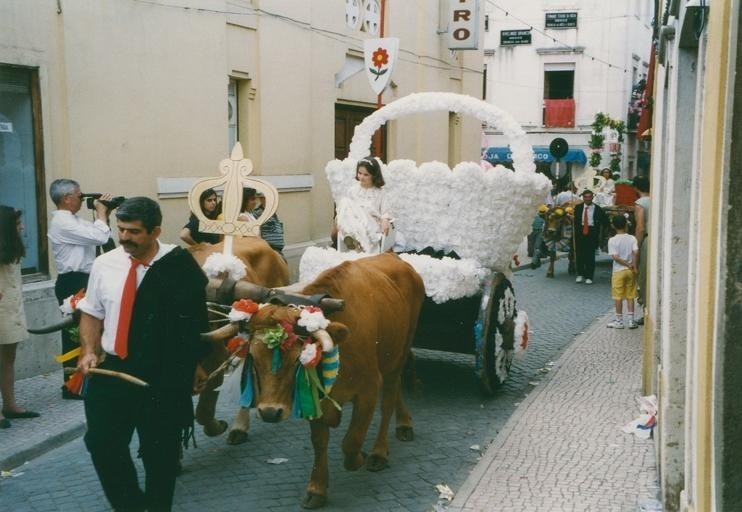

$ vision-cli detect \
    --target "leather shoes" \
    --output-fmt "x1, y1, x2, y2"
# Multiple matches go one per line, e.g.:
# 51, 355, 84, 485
0, 408, 39, 428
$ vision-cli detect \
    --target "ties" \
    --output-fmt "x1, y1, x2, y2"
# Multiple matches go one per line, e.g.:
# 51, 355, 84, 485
114, 259, 140, 360
583, 208, 589, 234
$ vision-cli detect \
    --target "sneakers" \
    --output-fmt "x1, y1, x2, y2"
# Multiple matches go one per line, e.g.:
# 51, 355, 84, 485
606, 317, 644, 328
576, 275, 592, 284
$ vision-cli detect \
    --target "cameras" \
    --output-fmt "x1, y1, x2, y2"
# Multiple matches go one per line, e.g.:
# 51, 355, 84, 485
81, 189, 125, 209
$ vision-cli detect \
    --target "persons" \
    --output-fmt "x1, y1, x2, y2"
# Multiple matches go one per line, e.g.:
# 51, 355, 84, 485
607, 215, 639, 329
1, 206, 40, 427
330, 156, 391, 250
180, 187, 286, 257
632, 173, 649, 324
49, 177, 112, 402
76, 198, 210, 510
528, 168, 616, 285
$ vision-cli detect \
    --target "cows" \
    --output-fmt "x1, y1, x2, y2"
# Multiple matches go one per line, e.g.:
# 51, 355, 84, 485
536, 199, 585, 277
26, 235, 292, 446
199, 251, 426, 511
